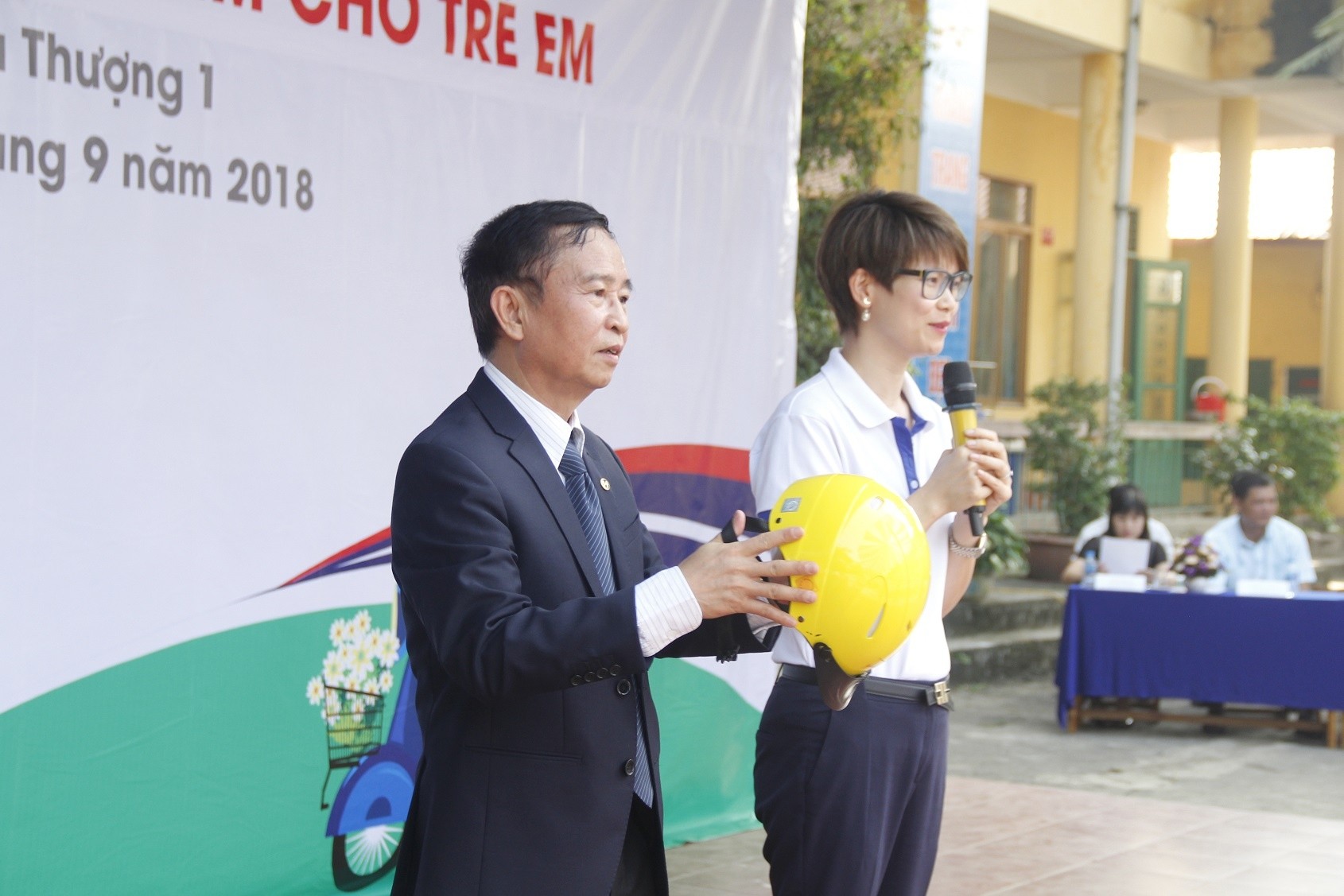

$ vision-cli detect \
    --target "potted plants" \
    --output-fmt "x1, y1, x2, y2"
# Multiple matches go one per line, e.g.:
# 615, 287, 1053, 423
1015, 377, 1134, 580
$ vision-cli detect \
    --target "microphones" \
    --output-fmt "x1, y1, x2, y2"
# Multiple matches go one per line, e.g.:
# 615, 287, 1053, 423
941, 361, 986, 536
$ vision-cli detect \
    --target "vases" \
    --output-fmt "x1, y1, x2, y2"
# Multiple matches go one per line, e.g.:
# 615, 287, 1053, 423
1184, 571, 1226, 593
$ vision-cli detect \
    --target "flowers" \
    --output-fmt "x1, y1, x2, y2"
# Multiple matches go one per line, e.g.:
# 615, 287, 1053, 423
1184, 537, 1218, 578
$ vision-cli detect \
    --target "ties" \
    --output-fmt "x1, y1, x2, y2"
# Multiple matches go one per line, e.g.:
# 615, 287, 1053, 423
558, 439, 654, 809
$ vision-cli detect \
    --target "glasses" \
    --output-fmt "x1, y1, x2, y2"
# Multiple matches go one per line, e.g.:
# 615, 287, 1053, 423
899, 269, 973, 301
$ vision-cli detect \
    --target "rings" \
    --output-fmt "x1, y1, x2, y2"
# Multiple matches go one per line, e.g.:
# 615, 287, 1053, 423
1001, 470, 1015, 478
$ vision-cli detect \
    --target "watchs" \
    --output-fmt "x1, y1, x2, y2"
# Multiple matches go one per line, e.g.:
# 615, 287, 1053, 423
947, 521, 988, 558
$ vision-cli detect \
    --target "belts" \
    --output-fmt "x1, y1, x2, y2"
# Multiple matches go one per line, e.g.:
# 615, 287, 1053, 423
777, 662, 950, 706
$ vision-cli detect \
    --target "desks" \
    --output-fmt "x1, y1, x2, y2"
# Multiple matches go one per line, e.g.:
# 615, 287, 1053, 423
1056, 581, 1344, 750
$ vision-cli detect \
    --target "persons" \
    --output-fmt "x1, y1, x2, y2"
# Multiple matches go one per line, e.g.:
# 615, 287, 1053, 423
391, 202, 818, 896
1196, 470, 1318, 590
1060, 483, 1177, 585
749, 192, 1014, 896
